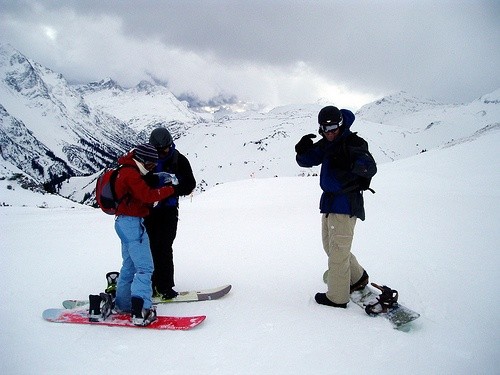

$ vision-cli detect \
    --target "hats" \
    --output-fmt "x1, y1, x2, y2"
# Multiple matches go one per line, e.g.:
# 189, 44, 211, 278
135, 143, 159, 166
318, 106, 344, 131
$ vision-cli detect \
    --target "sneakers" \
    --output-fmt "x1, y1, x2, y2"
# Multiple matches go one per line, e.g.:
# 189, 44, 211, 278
315, 292, 347, 308
133, 308, 157, 324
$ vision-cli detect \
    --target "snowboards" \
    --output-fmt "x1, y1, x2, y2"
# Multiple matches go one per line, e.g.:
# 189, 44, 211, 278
62, 285, 234, 312
323, 268, 422, 331
42, 307, 206, 331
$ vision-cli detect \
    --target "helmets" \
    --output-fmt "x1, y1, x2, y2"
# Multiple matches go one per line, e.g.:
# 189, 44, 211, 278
149, 128, 173, 150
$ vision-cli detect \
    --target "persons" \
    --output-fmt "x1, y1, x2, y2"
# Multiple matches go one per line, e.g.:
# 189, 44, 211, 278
110, 142, 177, 329
139, 126, 197, 303
294, 104, 379, 309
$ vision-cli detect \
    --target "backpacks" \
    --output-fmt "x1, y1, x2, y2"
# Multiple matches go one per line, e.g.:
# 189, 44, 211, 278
96, 163, 140, 216
